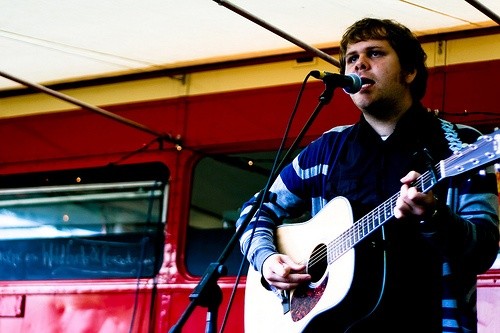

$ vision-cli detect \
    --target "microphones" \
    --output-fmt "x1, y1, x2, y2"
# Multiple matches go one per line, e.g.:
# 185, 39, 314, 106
310, 71, 362, 93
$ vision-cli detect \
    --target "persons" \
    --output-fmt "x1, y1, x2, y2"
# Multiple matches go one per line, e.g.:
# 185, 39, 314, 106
235, 16, 500, 333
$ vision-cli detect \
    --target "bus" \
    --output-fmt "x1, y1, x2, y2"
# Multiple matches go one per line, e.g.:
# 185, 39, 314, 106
0, 19, 500, 333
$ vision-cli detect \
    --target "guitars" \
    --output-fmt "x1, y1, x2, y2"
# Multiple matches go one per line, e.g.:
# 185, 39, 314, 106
242, 128, 500, 333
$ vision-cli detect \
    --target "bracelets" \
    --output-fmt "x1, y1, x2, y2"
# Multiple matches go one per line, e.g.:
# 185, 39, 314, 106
419, 196, 439, 224
